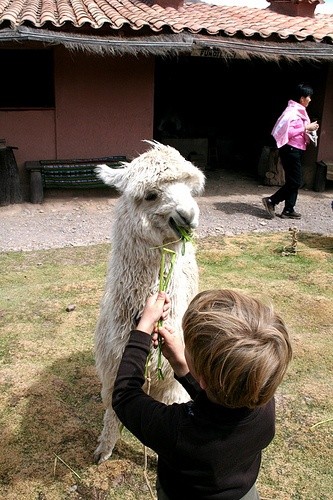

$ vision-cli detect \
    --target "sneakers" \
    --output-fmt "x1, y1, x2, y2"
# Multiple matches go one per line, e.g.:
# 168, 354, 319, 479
280, 207, 301, 219
261, 197, 275, 218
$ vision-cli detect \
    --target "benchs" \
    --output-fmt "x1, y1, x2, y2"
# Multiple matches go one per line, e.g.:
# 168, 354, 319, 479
25, 155, 132, 203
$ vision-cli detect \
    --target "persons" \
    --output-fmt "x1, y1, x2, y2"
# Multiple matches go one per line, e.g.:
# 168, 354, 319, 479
262, 83, 319, 219
111, 289, 293, 500
